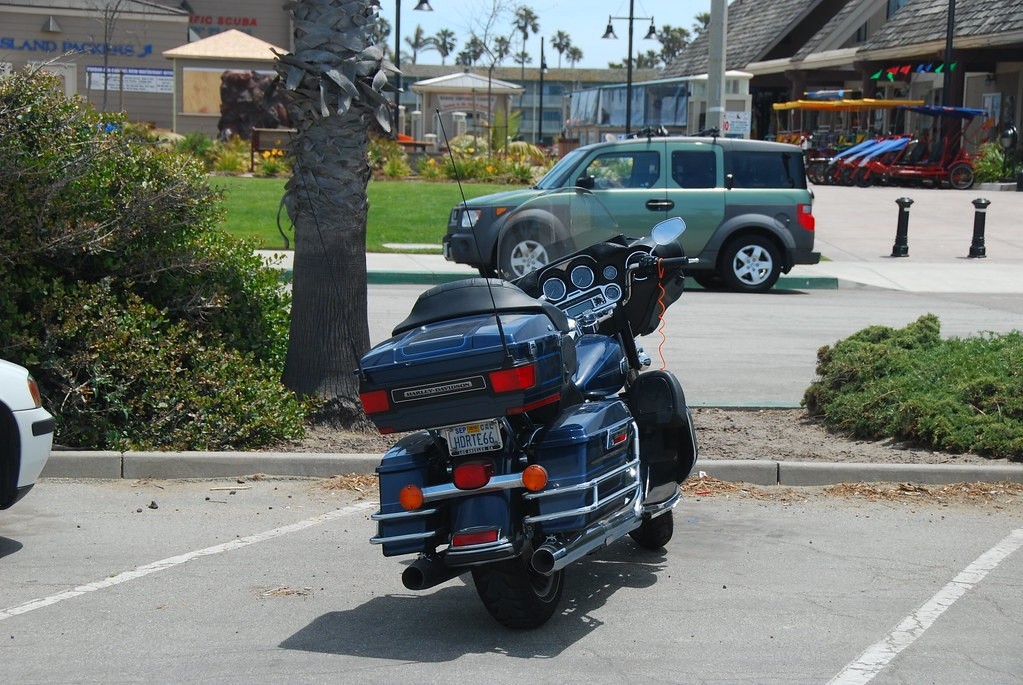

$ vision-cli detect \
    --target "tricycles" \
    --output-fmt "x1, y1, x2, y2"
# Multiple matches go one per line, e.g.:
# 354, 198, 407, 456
773, 89, 990, 192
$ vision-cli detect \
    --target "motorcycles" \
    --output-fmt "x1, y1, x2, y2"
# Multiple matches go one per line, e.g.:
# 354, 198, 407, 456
351, 185, 698, 633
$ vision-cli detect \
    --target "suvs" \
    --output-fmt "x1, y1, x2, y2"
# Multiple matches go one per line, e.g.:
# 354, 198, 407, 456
441, 125, 821, 298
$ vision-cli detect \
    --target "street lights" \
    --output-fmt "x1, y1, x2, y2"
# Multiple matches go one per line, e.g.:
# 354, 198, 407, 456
601, 0, 657, 138
537, 36, 548, 144
366, 0, 434, 137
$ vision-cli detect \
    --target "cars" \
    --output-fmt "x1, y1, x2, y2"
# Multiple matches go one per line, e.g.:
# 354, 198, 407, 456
0, 359, 57, 512
